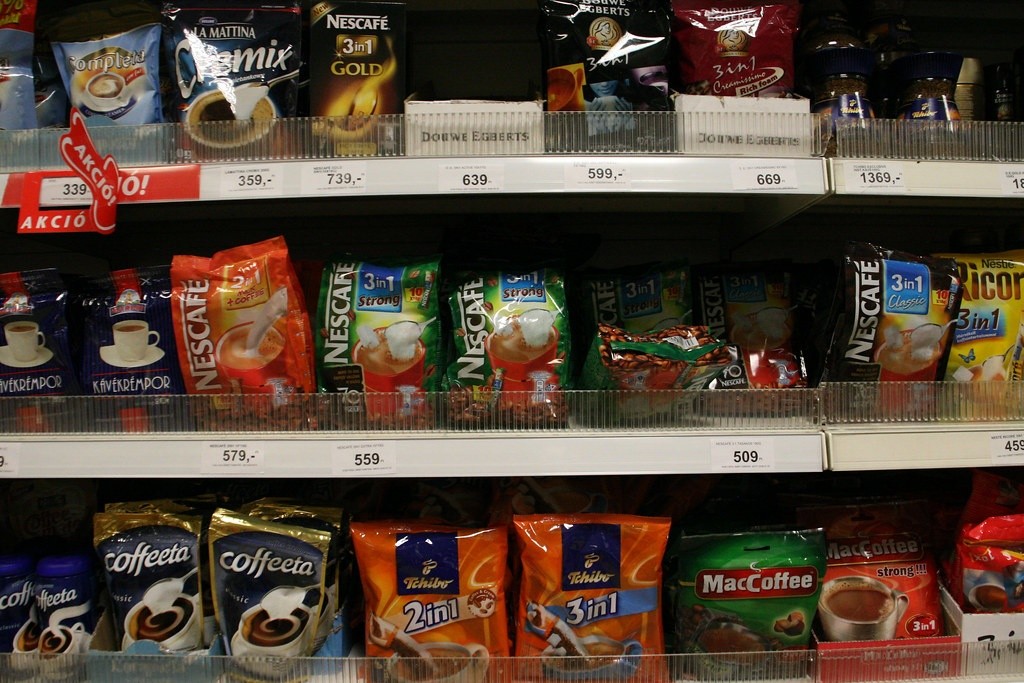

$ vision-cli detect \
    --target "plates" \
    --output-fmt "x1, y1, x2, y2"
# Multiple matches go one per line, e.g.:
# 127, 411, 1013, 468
0, 345, 53, 368
80, 92, 131, 112
99, 345, 165, 368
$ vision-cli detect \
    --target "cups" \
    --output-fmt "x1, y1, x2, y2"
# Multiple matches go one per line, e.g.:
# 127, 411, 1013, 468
961, 365, 1007, 391
85, 70, 127, 107
350, 327, 427, 425
40, 622, 86, 670
213, 321, 289, 411
485, 325, 560, 410
183, 89, 277, 160
817, 576, 910, 642
388, 642, 490, 683
547, 67, 584, 111
238, 603, 318, 656
541, 635, 643, 681
112, 320, 160, 361
4, 321, 47, 361
873, 329, 942, 415
124, 593, 199, 650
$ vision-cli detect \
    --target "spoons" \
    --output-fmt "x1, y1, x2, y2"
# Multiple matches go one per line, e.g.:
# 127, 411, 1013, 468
260, 584, 322, 611
910, 318, 955, 345
981, 344, 1015, 367
142, 568, 198, 603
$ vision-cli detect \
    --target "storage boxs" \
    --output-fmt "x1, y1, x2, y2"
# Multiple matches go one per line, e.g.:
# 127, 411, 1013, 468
810, 599, 963, 683
939, 577, 1024, 676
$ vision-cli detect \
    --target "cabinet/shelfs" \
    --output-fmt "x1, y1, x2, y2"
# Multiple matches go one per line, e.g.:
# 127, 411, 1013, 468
0, 112, 1024, 682
